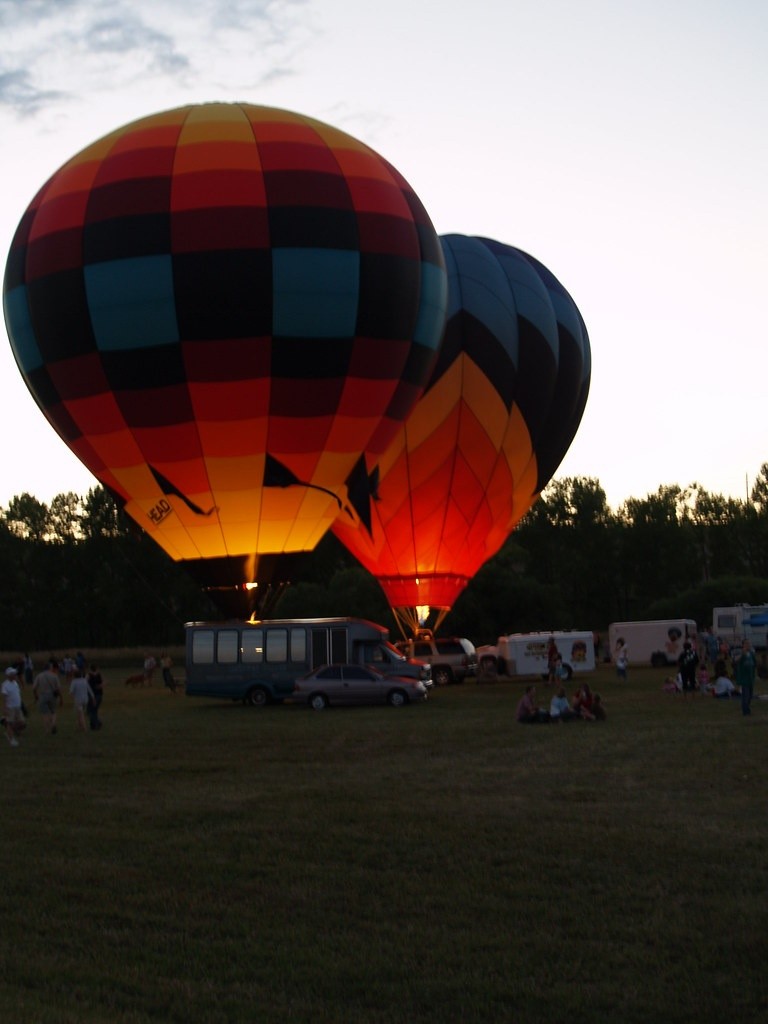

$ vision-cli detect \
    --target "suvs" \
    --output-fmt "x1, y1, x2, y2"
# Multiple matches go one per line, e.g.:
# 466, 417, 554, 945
392, 638, 478, 686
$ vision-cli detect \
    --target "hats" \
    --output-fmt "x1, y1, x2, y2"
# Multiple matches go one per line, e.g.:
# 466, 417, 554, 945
6, 667, 18, 675
684, 643, 691, 649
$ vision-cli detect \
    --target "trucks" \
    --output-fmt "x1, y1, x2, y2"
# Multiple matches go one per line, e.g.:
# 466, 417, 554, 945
184, 617, 408, 707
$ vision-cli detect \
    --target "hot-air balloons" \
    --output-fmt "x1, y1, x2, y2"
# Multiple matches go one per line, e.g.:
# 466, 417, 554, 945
328, 233, 593, 640
2, 101, 450, 624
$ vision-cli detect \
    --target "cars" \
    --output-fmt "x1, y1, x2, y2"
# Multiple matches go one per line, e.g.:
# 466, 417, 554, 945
291, 664, 428, 709
474, 643, 499, 670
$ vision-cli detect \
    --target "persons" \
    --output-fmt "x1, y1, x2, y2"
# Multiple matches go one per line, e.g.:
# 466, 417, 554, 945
49, 651, 74, 688
14, 653, 34, 688
75, 651, 87, 678
68, 662, 105, 731
143, 651, 158, 687
0, 666, 26, 746
160, 650, 174, 686
614, 638, 629, 686
31, 662, 63, 734
515, 683, 606, 724
677, 642, 698, 699
547, 653, 563, 685
695, 626, 734, 698
734, 638, 758, 716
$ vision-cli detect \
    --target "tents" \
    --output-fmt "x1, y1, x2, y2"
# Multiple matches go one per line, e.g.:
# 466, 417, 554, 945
741, 612, 768, 624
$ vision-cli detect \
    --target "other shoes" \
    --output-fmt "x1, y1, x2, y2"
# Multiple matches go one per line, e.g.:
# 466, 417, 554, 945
11, 741, 19, 747
91, 721, 103, 730
51, 726, 56, 735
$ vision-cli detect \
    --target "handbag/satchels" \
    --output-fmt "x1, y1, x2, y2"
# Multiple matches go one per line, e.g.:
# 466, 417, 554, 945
21, 704, 29, 717
52, 689, 60, 698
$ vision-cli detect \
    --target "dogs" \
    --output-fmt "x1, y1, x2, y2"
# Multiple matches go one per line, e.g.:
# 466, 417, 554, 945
0, 716, 26, 736
126, 674, 147, 688
